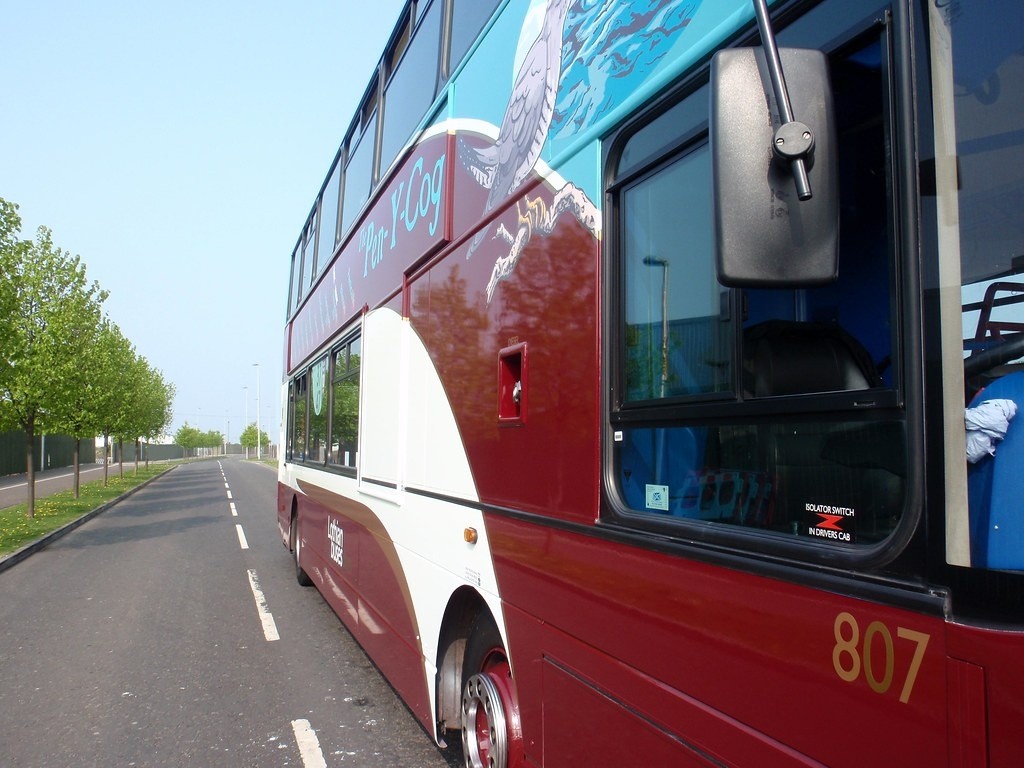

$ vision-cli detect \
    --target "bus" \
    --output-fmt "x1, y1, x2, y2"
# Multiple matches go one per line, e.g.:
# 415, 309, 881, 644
269, 1, 1024, 767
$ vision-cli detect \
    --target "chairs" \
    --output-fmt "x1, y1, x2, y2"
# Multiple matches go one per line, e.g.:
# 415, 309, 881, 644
620, 364, 1024, 571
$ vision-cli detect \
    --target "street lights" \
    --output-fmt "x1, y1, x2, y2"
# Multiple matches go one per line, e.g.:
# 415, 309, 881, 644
241, 385, 250, 459
228, 421, 231, 443
252, 362, 260, 460
642, 252, 670, 484
